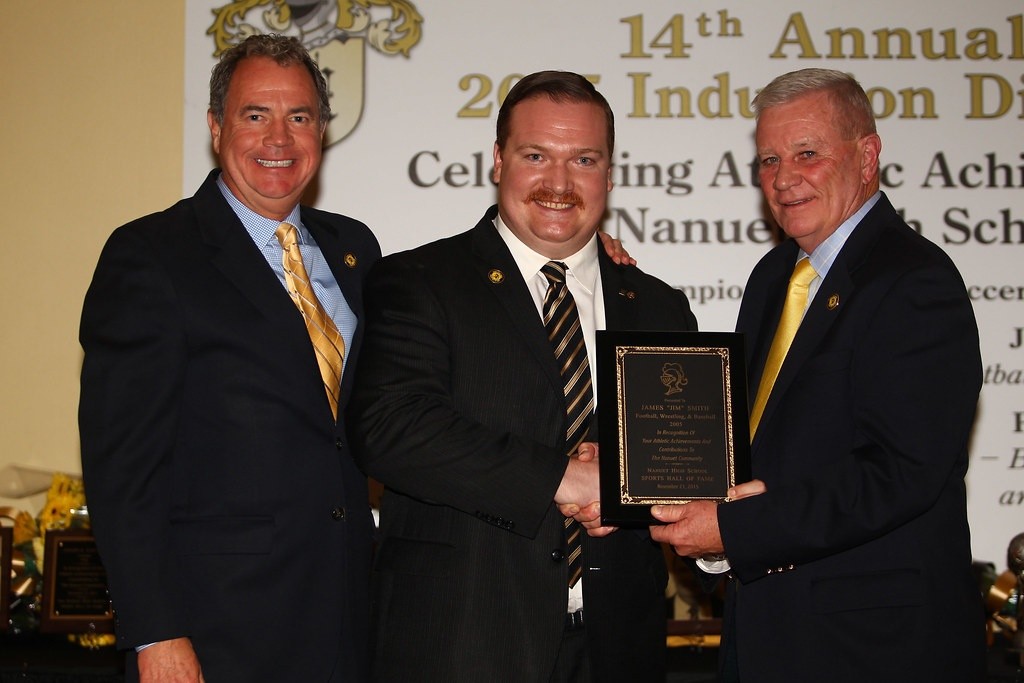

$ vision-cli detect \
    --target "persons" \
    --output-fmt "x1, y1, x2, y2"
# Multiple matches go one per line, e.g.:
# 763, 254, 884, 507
78, 35, 637, 682
557, 68, 986, 682
345, 71, 697, 682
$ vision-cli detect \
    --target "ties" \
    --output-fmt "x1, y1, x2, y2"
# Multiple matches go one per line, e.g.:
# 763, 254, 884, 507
274, 221, 345, 427
540, 261, 599, 589
747, 257, 821, 446
